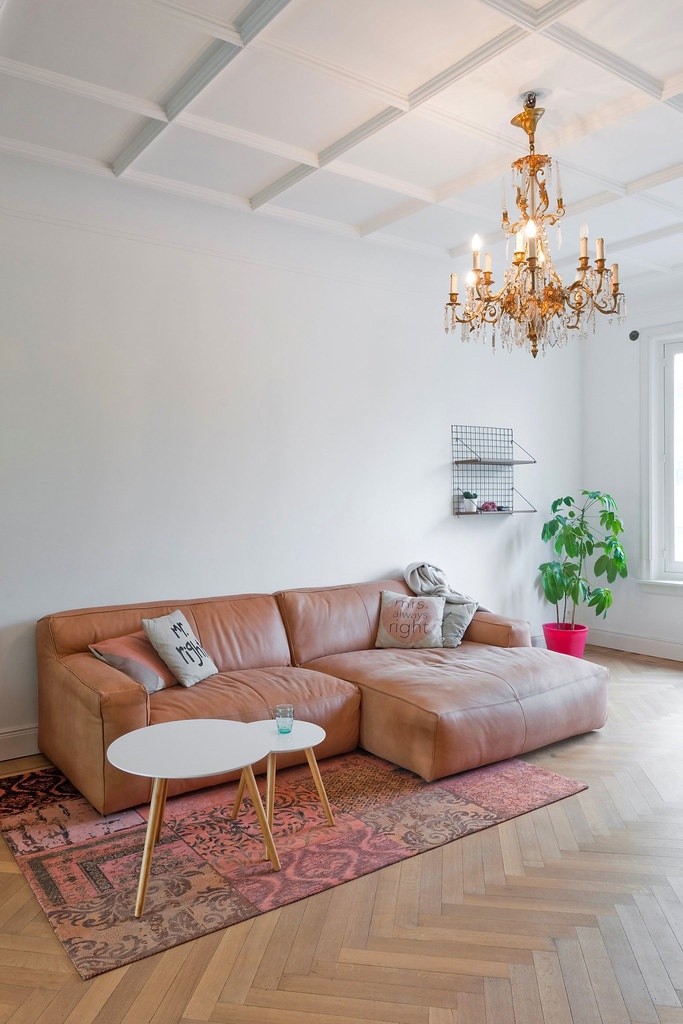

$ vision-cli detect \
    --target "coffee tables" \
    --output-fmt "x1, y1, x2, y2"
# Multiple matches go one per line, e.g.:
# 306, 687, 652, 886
231, 717, 336, 859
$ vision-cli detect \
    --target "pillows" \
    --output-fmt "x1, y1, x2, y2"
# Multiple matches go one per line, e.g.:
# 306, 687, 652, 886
375, 589, 446, 648
88, 629, 181, 696
441, 601, 479, 648
142, 610, 219, 688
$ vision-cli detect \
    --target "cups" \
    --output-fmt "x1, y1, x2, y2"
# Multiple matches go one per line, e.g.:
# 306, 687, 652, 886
276, 704, 294, 733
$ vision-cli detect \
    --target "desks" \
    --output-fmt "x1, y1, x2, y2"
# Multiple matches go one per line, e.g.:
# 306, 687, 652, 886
107, 719, 280, 920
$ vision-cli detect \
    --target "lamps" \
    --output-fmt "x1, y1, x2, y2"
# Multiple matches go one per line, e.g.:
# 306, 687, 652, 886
444, 91, 627, 359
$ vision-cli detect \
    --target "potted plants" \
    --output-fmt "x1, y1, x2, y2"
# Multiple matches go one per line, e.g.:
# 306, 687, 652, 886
462, 492, 478, 513
539, 489, 628, 658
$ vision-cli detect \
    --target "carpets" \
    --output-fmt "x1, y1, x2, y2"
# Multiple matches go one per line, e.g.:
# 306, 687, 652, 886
1, 757, 589, 980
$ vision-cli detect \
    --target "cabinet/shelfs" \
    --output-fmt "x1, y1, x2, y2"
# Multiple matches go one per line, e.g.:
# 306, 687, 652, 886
451, 425, 538, 516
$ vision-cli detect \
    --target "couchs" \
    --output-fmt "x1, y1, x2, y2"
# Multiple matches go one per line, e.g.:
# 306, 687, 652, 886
37, 579, 610, 815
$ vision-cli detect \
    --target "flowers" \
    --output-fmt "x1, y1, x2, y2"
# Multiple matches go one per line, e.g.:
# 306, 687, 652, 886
482, 502, 497, 511
